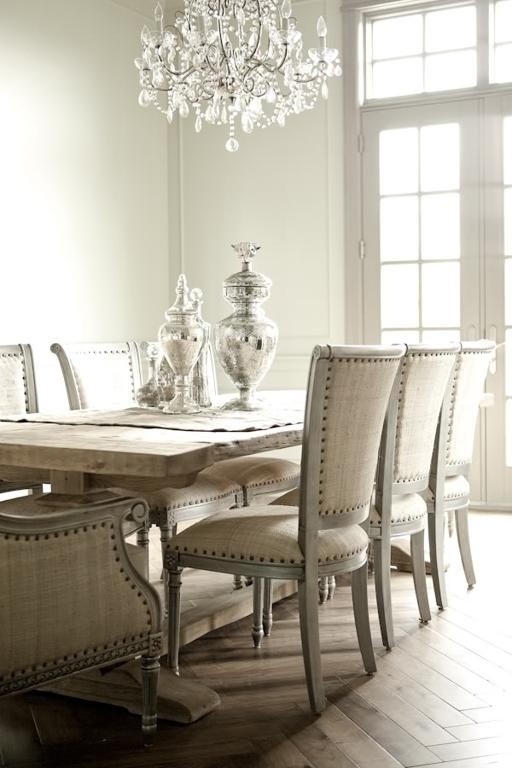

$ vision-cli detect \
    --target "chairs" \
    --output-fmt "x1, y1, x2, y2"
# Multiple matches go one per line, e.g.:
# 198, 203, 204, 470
263, 344, 461, 649
51, 341, 244, 619
168, 343, 407, 714
0, 344, 43, 495
0, 497, 163, 749
328, 339, 496, 609
135, 338, 302, 587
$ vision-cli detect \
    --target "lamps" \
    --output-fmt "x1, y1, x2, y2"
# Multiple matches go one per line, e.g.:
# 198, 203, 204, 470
134, 1, 343, 153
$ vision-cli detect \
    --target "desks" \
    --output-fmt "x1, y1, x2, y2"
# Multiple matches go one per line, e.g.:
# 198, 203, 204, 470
0, 388, 492, 724
